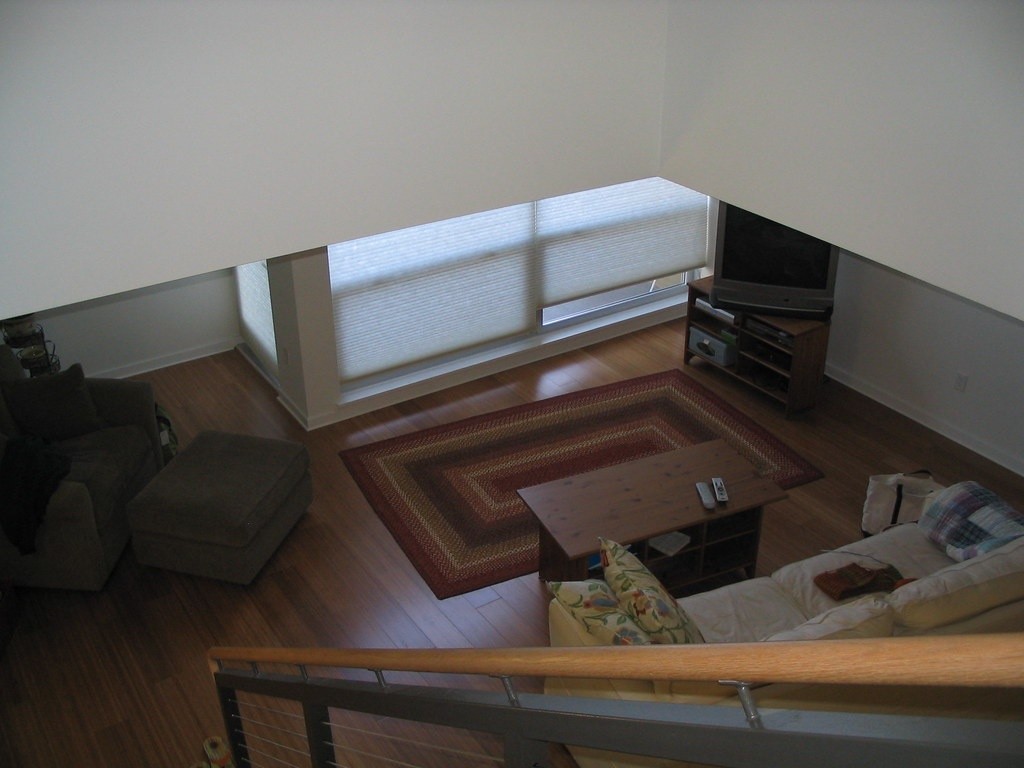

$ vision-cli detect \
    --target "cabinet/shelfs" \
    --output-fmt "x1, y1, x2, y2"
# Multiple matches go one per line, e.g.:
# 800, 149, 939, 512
684, 275, 833, 418
514, 438, 789, 593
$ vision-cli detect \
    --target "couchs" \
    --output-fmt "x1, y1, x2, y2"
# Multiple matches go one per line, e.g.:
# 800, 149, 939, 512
0, 345, 165, 588
547, 479, 1024, 768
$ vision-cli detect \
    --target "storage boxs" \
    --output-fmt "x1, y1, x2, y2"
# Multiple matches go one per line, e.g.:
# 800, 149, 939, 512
688, 326, 735, 367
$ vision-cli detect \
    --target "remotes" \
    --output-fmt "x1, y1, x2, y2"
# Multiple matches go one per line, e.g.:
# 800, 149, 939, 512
696, 482, 715, 510
712, 478, 729, 502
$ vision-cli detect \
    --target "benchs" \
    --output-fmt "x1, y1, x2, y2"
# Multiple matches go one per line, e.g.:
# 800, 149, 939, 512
126, 428, 314, 585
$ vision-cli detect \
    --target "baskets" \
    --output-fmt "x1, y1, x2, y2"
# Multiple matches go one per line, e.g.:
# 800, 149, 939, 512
30, 340, 61, 377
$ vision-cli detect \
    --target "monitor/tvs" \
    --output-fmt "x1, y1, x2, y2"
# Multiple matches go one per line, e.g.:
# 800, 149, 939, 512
709, 200, 840, 319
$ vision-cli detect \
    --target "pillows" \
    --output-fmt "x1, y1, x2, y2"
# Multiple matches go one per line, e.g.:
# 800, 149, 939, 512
0, 435, 71, 554
593, 534, 715, 645
0, 362, 107, 443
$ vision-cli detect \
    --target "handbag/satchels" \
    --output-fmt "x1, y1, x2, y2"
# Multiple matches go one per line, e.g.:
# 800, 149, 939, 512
861, 470, 947, 538
154, 402, 179, 463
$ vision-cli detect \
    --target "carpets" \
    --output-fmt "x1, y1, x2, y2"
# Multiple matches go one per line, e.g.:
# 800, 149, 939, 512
339, 370, 826, 602
